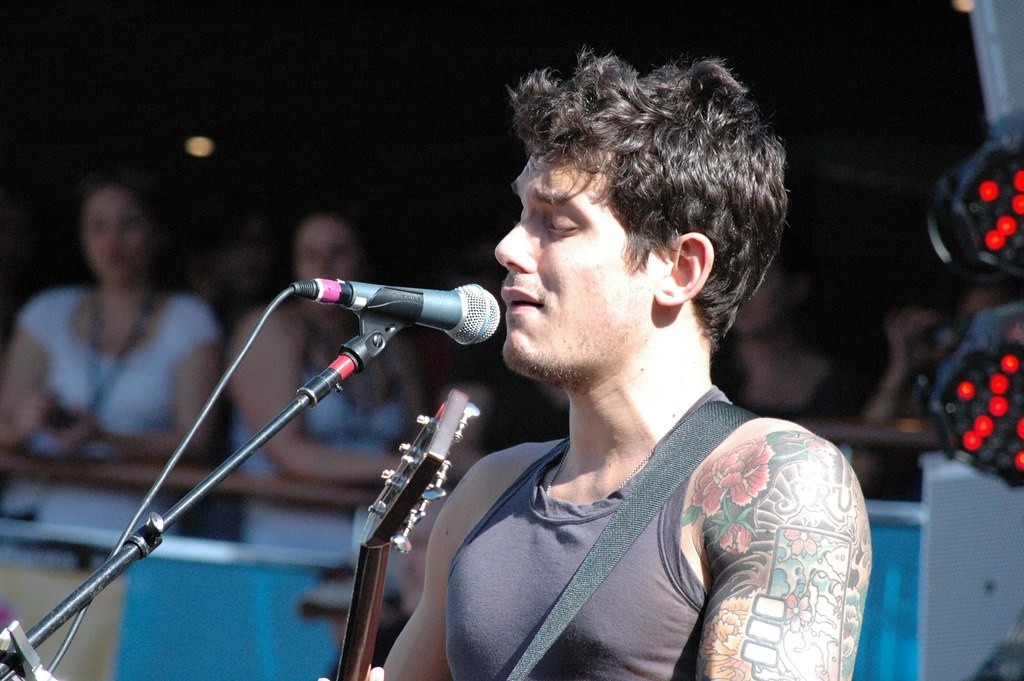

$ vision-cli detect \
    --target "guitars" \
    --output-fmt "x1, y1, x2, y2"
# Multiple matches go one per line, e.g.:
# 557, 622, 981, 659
334, 387, 481, 681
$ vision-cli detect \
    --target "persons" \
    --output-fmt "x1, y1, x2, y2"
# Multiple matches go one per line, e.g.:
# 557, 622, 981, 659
724, 232, 1020, 503
320, 51, 872, 681
0, 167, 562, 545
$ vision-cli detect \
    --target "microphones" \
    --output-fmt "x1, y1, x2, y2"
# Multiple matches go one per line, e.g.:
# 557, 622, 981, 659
293, 276, 501, 346
